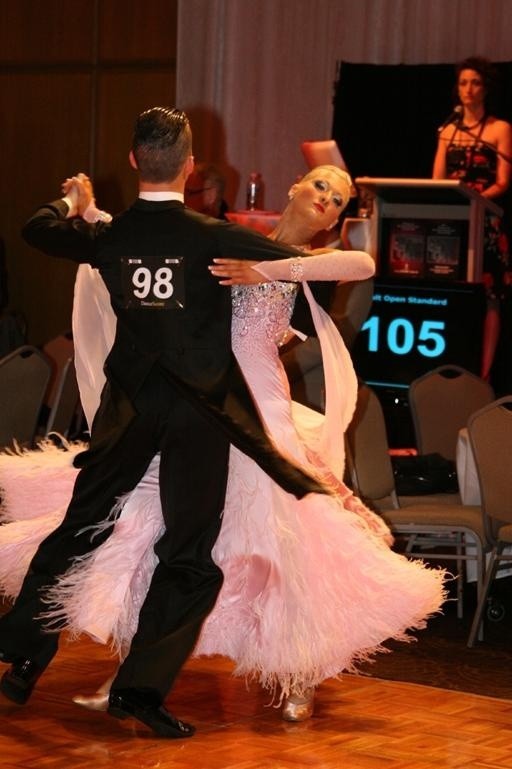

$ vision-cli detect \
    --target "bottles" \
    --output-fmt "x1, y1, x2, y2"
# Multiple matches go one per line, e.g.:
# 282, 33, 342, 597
245, 172, 260, 212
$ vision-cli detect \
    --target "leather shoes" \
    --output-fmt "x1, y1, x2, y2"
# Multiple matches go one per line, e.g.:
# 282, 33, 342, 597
282, 696, 314, 721
0, 641, 58, 704
71, 689, 196, 737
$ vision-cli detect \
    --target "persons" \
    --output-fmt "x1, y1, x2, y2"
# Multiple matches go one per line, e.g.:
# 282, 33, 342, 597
0, 164, 457, 724
0, 106, 337, 737
181, 162, 233, 222
430, 56, 512, 384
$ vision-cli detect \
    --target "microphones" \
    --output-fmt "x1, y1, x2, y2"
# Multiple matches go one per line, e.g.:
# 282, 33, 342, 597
437, 105, 463, 133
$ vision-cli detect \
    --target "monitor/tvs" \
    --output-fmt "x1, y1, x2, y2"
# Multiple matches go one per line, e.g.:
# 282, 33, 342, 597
351, 273, 486, 393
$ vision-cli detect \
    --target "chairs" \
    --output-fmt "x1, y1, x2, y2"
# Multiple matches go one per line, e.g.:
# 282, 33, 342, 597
0, 328, 89, 449
343, 365, 511, 651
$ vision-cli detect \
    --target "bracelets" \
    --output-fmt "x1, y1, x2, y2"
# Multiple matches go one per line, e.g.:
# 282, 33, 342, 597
290, 259, 304, 284
